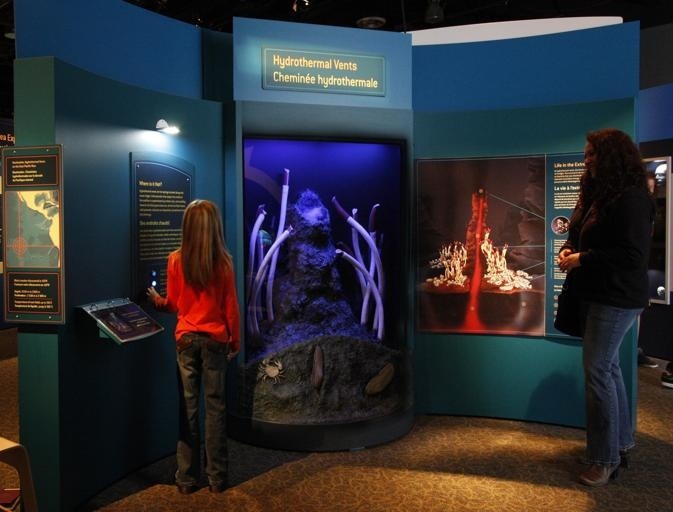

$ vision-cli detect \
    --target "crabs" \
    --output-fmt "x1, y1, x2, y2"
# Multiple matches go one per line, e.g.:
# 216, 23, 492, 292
258, 358, 287, 385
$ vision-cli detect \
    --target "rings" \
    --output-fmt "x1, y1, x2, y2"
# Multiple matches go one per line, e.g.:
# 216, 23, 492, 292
563, 263, 567, 268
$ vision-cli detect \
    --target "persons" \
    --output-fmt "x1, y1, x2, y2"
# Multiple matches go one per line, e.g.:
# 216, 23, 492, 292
653, 163, 667, 189
552, 220, 568, 234
145, 199, 241, 495
633, 172, 658, 369
659, 359, 672, 389
109, 311, 129, 333
554, 126, 658, 488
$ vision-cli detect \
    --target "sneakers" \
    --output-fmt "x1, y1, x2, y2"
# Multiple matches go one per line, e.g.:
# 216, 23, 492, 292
637, 352, 659, 370
660, 371, 673, 390
619, 446, 633, 469
579, 459, 621, 487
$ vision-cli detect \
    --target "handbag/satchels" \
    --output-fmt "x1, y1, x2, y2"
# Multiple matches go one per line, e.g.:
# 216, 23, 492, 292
553, 267, 588, 339
